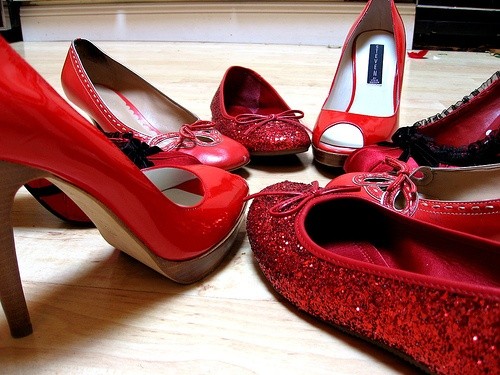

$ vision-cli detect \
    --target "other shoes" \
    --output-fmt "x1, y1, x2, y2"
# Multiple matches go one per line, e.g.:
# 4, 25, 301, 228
23, 134, 202, 225
252, 180, 499, 375
344, 71, 500, 176
211, 66, 311, 158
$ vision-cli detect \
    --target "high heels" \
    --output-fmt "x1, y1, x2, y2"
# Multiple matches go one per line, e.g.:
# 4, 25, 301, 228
313, 0, 406, 168
0, 36, 249, 340
326, 169, 500, 244
63, 38, 251, 172
